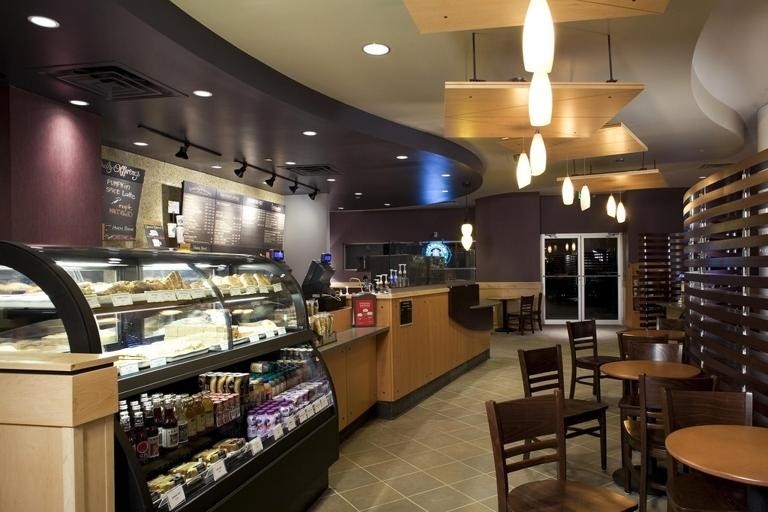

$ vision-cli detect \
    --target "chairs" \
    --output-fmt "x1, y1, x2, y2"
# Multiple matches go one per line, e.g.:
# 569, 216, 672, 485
563, 317, 627, 402
507, 294, 535, 337
598, 325, 768, 511
514, 343, 609, 472
521, 292, 544, 331
484, 387, 639, 511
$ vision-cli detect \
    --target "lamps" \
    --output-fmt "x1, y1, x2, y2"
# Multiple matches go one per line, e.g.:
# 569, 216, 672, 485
459, 180, 473, 237
231, 157, 321, 201
173, 135, 191, 160
510, 0, 628, 225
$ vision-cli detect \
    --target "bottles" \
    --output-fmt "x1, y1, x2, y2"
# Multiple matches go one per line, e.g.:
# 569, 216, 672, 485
247, 359, 330, 439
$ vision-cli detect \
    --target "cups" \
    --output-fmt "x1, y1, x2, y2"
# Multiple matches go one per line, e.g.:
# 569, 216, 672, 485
166, 223, 176, 239
175, 227, 185, 244
176, 215, 185, 226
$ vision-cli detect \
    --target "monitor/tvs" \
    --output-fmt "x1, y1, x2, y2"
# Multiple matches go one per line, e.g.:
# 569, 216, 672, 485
301, 260, 346, 309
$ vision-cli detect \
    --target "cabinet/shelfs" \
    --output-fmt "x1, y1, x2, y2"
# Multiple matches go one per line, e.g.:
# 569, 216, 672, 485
624, 259, 670, 329
0, 239, 340, 511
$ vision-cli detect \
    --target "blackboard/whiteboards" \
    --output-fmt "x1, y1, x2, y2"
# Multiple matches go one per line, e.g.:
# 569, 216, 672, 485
182, 181, 285, 251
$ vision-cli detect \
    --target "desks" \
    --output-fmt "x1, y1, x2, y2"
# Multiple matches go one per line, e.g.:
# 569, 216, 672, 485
483, 294, 522, 332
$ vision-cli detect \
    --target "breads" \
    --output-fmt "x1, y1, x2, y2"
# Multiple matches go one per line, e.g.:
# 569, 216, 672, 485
0, 269, 278, 374
